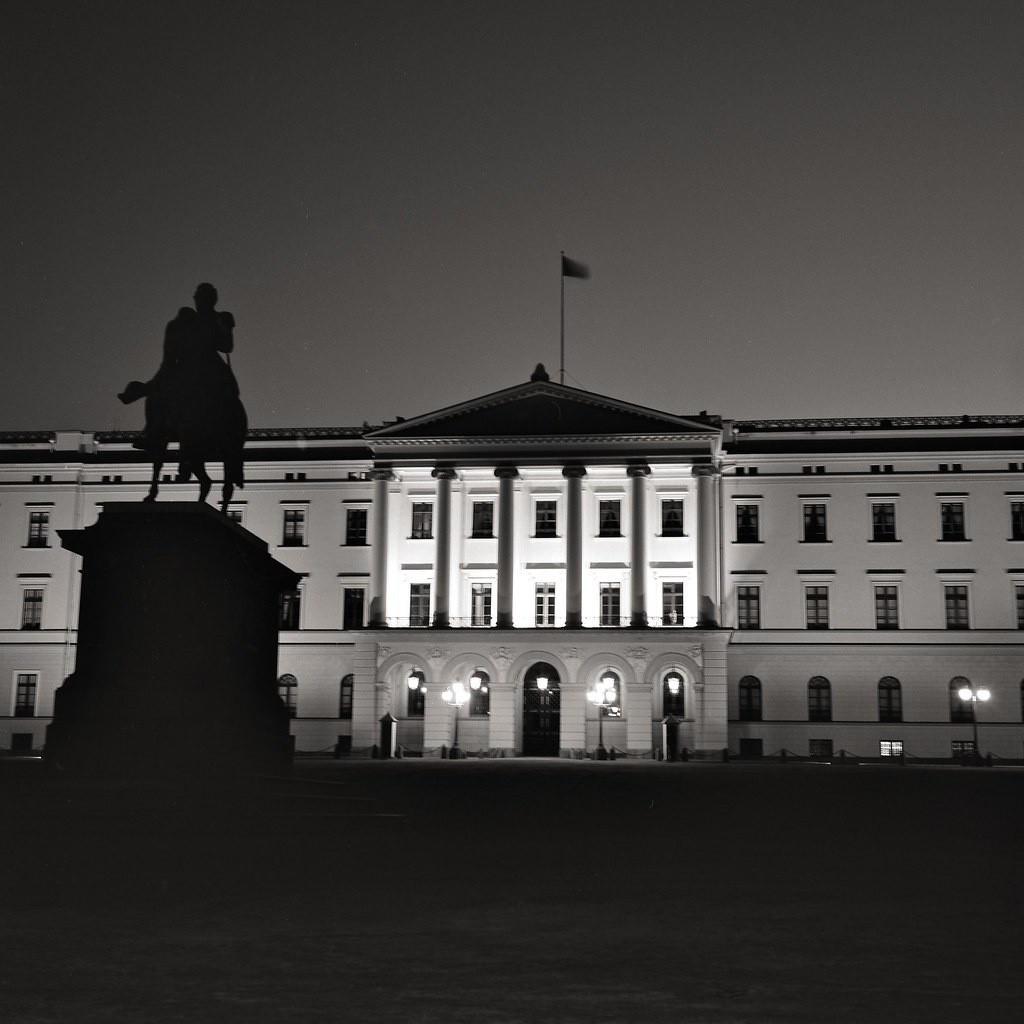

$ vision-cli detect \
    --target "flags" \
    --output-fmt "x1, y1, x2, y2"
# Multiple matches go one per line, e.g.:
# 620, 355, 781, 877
563, 255, 589, 278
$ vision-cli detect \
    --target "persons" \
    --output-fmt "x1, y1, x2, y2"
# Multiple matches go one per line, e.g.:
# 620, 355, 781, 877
131, 284, 241, 454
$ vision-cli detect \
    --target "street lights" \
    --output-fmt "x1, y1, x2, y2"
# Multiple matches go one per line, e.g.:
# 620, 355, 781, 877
442, 681, 471, 760
958, 683, 992, 767
651, 677, 696, 763
586, 677, 618, 759
